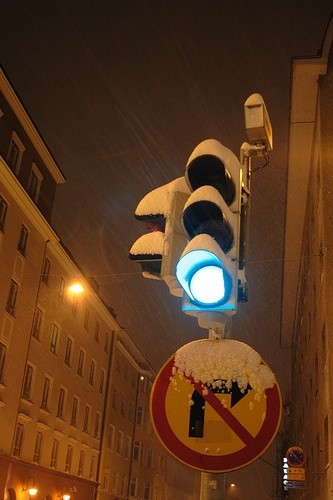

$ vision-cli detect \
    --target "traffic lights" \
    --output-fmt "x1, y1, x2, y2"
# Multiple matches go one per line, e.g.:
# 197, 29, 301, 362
130, 176, 189, 297
176, 139, 242, 319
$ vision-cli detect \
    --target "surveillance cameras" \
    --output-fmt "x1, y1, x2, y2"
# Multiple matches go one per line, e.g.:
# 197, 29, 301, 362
243, 93, 274, 151
